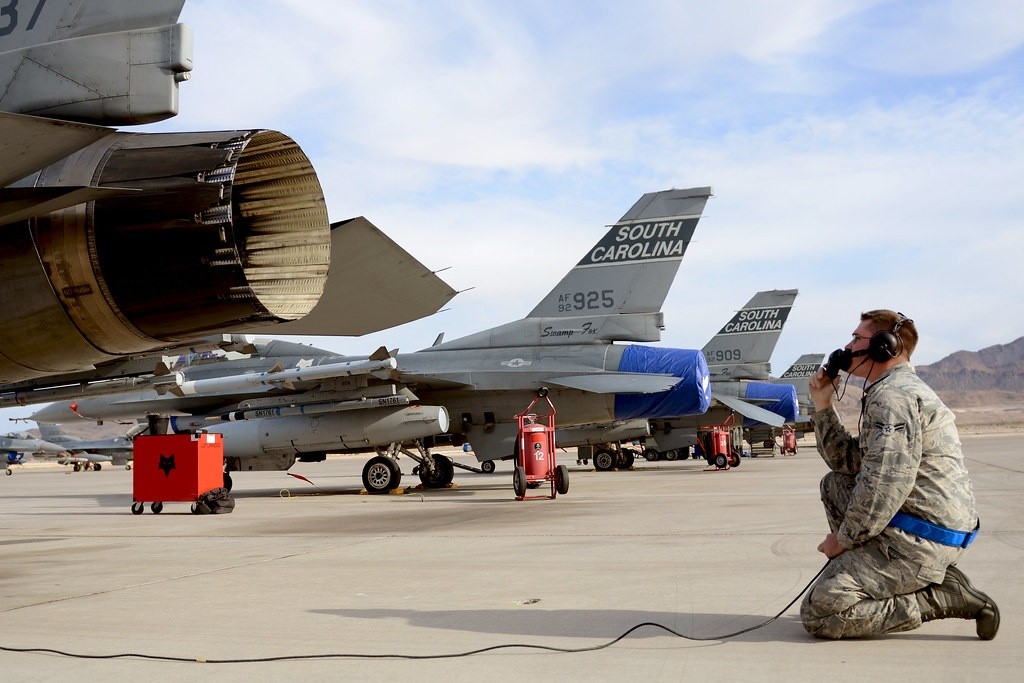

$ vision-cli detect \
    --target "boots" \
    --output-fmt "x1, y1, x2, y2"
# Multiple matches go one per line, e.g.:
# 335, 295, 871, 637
921, 564, 1000, 640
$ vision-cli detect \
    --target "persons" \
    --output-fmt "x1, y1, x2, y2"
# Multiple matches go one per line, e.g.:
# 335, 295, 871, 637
799, 309, 1001, 641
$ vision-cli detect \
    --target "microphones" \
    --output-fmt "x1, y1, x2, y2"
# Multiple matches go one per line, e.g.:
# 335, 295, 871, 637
841, 349, 868, 371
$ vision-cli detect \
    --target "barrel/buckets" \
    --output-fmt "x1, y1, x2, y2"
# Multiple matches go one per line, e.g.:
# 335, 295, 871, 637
146, 413, 170, 435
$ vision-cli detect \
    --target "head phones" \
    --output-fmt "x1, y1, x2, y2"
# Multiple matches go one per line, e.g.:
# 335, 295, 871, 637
867, 316, 913, 364
